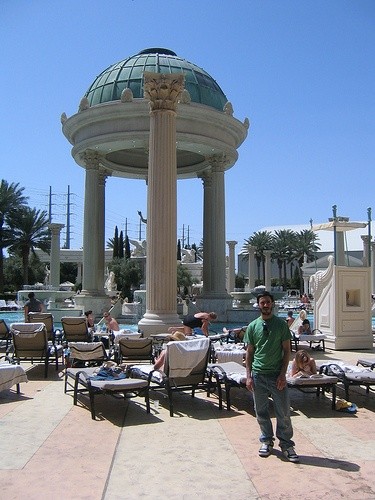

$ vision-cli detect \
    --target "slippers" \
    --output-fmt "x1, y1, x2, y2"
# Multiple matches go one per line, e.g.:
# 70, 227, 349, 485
337, 401, 358, 413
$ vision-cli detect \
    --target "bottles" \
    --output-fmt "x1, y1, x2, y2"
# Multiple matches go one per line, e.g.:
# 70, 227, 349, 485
102, 323, 106, 333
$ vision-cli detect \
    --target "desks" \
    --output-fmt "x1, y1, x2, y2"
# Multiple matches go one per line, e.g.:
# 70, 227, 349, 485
112, 330, 143, 346
92, 332, 112, 342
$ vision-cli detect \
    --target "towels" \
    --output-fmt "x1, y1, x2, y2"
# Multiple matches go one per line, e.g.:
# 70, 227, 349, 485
337, 364, 373, 373
68, 342, 103, 360
295, 334, 327, 341
10, 323, 45, 336
230, 372, 247, 384
216, 351, 243, 366
0, 359, 28, 393
166, 338, 210, 378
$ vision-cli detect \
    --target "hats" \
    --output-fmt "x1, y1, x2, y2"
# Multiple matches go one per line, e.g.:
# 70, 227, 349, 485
165, 330, 186, 342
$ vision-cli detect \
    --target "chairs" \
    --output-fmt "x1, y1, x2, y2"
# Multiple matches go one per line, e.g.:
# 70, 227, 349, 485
127, 338, 222, 417
227, 326, 248, 343
64, 367, 150, 420
0, 356, 28, 393
0, 319, 13, 350
118, 337, 155, 365
27, 311, 62, 345
315, 360, 375, 399
356, 359, 375, 370
290, 329, 327, 352
61, 316, 93, 343
207, 362, 252, 410
211, 341, 247, 366
68, 342, 117, 366
10, 323, 64, 378
286, 365, 338, 407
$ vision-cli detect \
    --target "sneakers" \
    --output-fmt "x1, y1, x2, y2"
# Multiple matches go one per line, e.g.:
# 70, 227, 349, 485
283, 445, 300, 464
258, 439, 271, 457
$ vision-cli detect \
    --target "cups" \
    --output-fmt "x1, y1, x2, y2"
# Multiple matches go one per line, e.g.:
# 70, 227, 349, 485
97, 326, 100, 333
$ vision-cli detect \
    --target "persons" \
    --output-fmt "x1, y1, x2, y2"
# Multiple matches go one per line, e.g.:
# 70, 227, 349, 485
286, 311, 295, 327
168, 312, 218, 337
111, 293, 128, 305
298, 319, 312, 335
24, 292, 44, 323
285, 349, 317, 379
243, 291, 301, 463
104, 311, 120, 332
153, 331, 186, 378
301, 292, 311, 303
85, 311, 95, 329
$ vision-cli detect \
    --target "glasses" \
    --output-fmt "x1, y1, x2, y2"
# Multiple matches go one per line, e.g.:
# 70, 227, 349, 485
104, 315, 109, 317
